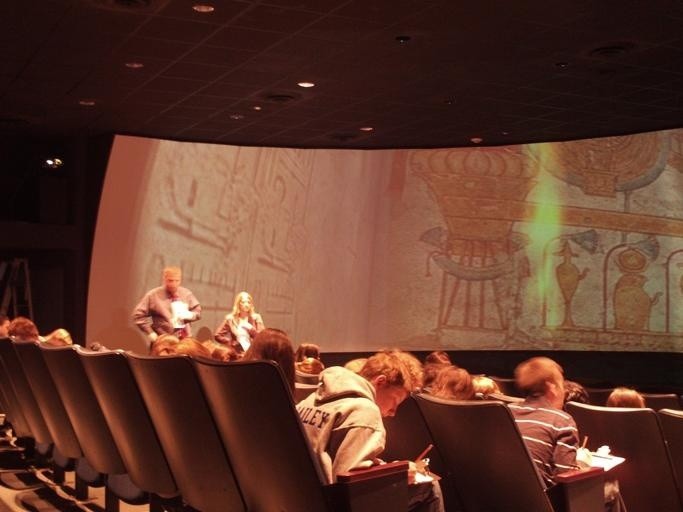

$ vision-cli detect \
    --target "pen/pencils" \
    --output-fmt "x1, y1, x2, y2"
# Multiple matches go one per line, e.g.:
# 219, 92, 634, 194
581, 436, 588, 448
414, 444, 434, 463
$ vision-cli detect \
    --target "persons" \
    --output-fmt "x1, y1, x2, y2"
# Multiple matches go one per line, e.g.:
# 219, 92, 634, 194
1, 267, 645, 511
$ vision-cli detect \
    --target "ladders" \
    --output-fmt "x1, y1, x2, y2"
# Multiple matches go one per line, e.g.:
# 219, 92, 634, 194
0, 257, 34, 322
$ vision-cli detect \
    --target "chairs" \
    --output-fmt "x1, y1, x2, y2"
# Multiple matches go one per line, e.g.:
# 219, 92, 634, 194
1, 334, 683, 512
